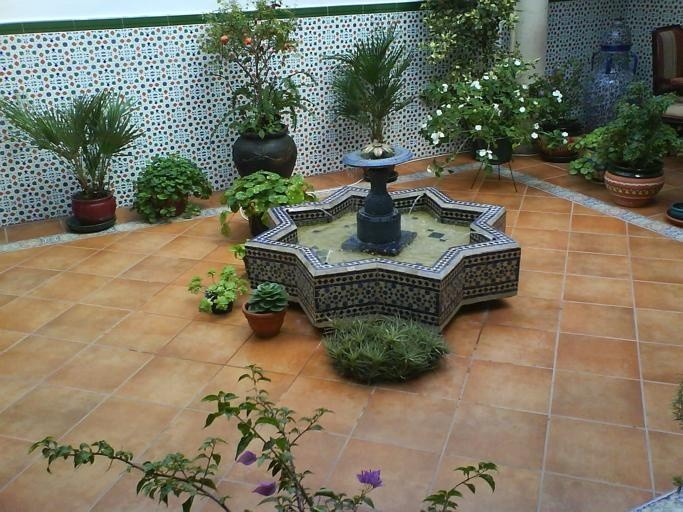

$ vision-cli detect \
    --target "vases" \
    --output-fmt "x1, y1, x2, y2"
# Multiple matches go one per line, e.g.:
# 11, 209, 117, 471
470, 136, 518, 192
670, 203, 683, 220
665, 207, 683, 227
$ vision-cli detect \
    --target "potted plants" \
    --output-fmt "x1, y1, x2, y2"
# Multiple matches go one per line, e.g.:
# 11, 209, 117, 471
568, 128, 607, 186
232, 241, 248, 272
242, 282, 289, 337
0, 89, 147, 225
328, 24, 416, 179
135, 156, 214, 224
528, 59, 586, 162
529, 57, 594, 162
200, 0, 319, 178
595, 82, 683, 207
219, 169, 320, 236
187, 266, 248, 314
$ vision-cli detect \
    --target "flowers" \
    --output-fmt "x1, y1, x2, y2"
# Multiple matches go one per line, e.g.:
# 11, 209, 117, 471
418, 56, 568, 178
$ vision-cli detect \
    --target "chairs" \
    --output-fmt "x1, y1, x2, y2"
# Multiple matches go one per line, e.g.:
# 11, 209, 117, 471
652, 25, 683, 135
